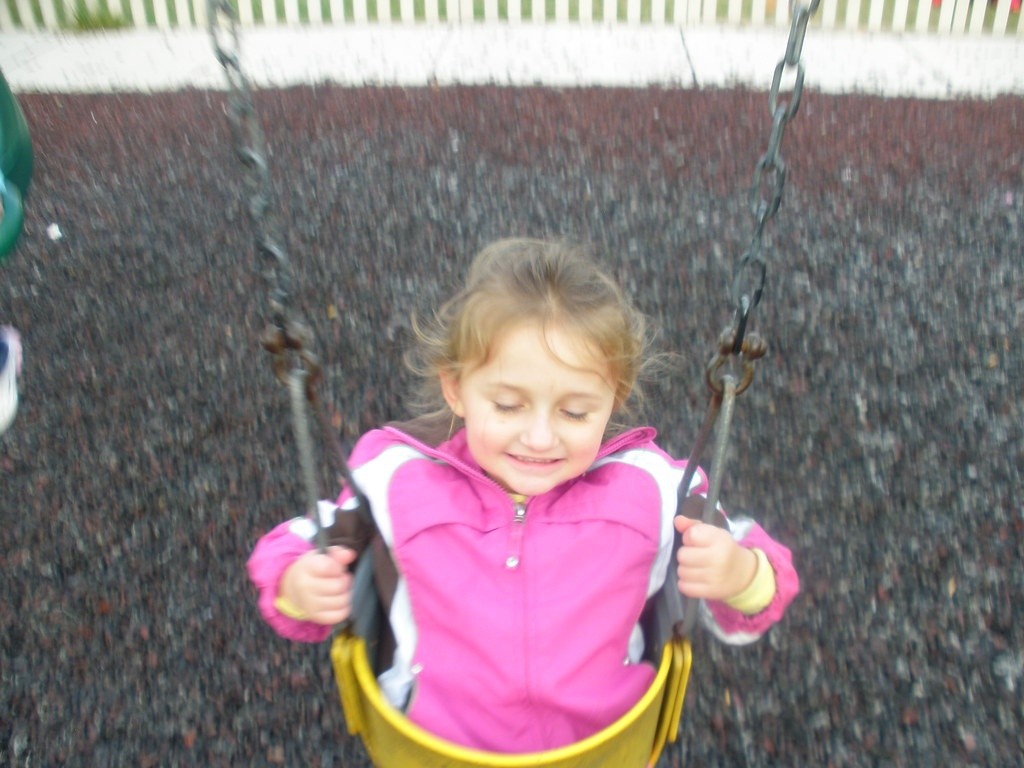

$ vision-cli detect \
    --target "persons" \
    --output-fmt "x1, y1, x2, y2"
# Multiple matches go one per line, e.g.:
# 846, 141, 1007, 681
246, 238, 799, 756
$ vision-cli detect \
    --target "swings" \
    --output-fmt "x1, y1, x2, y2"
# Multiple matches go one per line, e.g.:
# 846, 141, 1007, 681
206, 0, 824, 768
